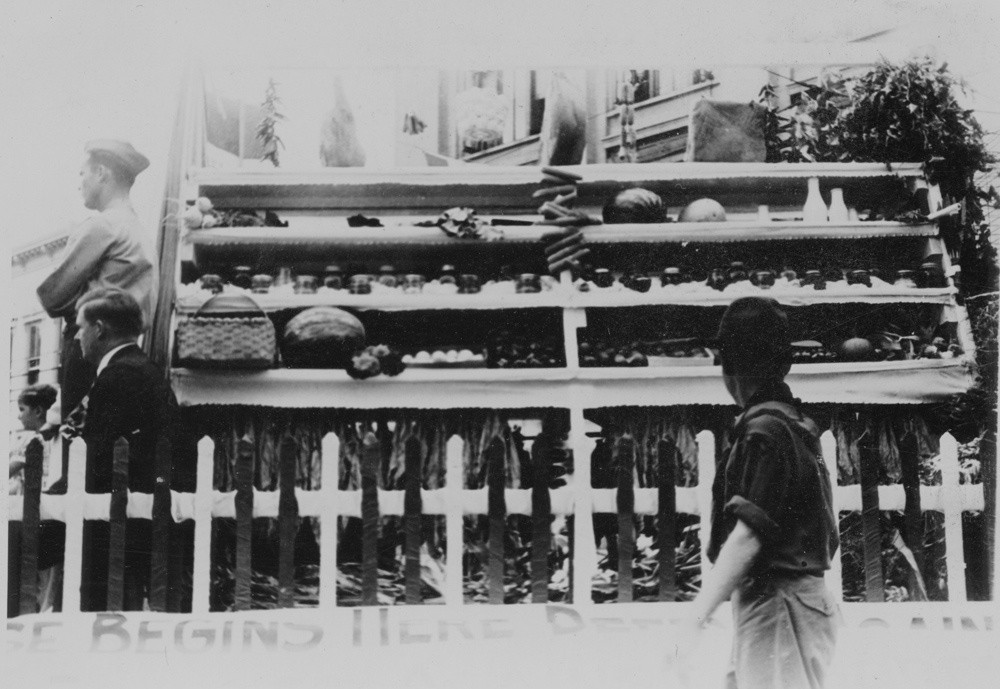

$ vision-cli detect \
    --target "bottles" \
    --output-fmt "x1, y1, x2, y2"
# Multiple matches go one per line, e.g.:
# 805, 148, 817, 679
757, 204, 772, 221
802, 178, 848, 224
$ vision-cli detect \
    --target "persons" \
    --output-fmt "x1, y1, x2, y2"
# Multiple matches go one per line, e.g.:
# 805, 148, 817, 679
37, 134, 152, 493
681, 294, 840, 689
8, 385, 73, 616
69, 285, 180, 612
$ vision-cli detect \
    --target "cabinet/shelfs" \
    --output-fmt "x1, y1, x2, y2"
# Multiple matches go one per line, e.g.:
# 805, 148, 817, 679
154, 163, 976, 413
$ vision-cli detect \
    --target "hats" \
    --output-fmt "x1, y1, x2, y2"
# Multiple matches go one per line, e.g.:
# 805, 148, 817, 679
17, 384, 59, 408
83, 139, 149, 183
711, 296, 790, 359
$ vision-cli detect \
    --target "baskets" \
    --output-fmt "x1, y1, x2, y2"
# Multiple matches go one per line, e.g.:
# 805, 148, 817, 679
176, 315, 276, 368
